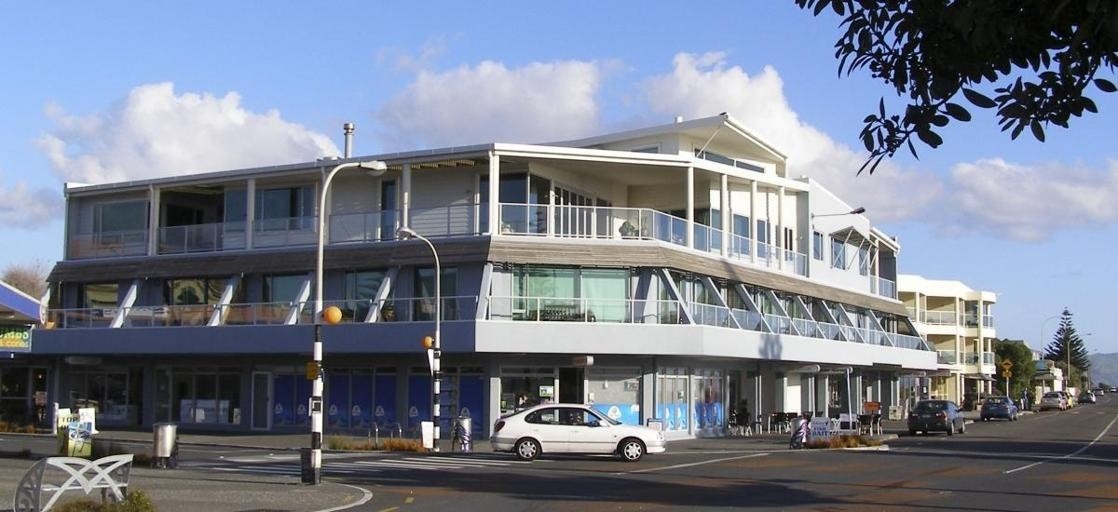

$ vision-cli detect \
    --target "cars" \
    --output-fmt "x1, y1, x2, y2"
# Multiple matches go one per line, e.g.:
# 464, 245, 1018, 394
490, 402, 667, 462
980, 395, 1018, 422
1040, 385, 1117, 411
69, 391, 99, 415
908, 398, 966, 436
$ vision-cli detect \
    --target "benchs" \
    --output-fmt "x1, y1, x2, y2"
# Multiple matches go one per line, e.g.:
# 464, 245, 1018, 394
13, 453, 135, 512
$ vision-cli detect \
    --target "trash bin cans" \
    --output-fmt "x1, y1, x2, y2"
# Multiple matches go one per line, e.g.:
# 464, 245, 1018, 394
790, 418, 808, 449
452, 417, 472, 453
153, 422, 179, 470
300, 448, 322, 484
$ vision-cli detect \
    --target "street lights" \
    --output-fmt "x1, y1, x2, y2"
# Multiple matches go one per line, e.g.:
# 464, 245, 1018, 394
1088, 348, 1099, 391
310, 160, 389, 489
1041, 313, 1071, 397
397, 221, 442, 454
1067, 333, 1092, 382
